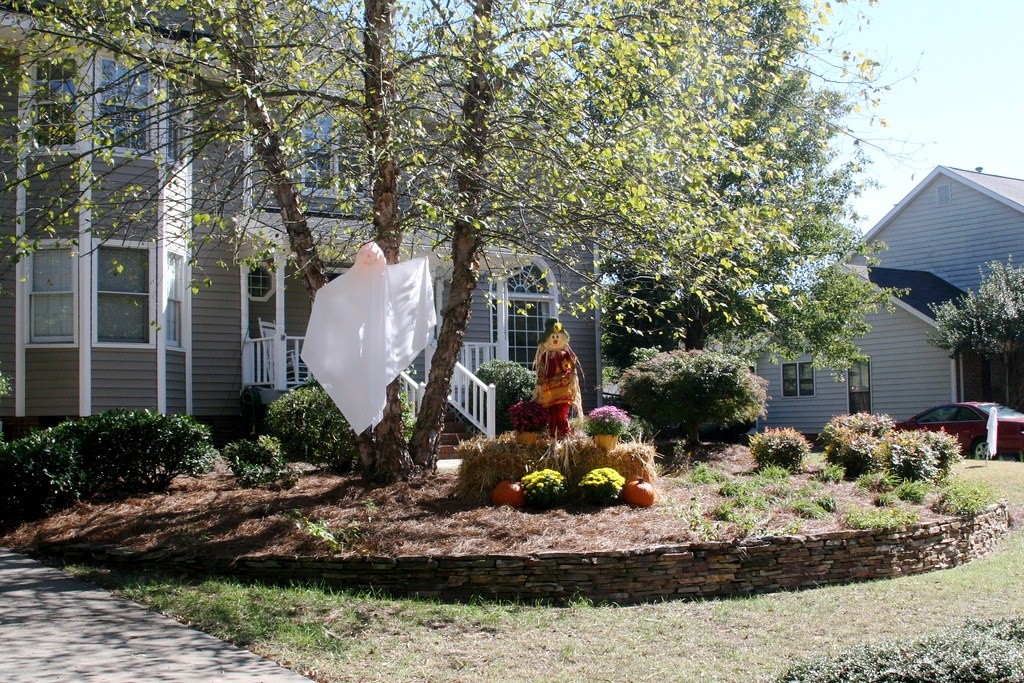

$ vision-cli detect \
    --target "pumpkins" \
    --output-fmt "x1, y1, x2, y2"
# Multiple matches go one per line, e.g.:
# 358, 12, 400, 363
491, 476, 526, 508
623, 477, 654, 508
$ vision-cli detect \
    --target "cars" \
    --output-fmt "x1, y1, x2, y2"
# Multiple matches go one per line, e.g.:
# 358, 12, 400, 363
893, 401, 1024, 459
